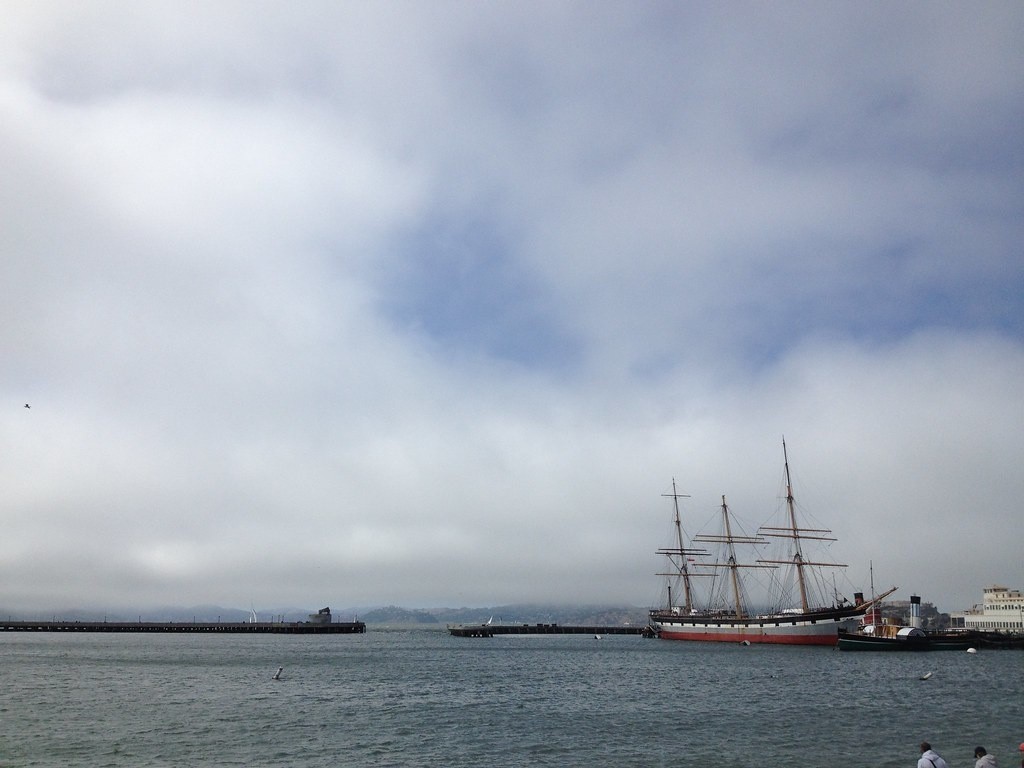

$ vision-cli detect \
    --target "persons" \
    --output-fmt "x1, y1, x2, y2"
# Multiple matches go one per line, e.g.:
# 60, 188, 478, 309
974, 747, 1000, 767
917, 742, 950, 767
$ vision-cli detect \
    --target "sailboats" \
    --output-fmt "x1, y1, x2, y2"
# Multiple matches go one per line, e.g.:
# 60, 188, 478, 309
647, 431, 900, 646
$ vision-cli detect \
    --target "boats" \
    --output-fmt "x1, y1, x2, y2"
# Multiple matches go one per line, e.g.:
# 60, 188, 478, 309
837, 627, 980, 651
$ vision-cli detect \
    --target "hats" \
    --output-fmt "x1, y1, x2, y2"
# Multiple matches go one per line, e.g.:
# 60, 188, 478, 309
1019, 742, 1024, 751
974, 747, 984, 758
